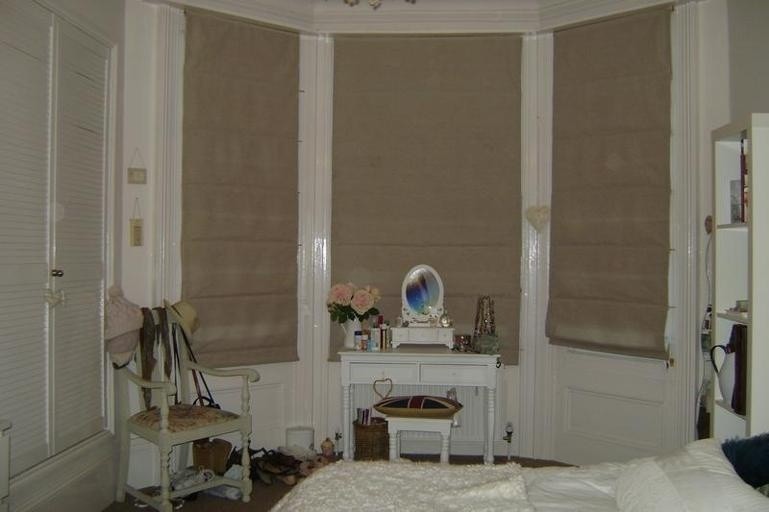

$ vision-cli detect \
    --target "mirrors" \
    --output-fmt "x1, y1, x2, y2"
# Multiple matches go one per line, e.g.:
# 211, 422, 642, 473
402, 263, 445, 327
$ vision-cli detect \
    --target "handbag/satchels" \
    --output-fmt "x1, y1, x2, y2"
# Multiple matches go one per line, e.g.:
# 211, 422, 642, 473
258, 453, 298, 474
193, 397, 220, 409
174, 466, 215, 491
193, 438, 232, 473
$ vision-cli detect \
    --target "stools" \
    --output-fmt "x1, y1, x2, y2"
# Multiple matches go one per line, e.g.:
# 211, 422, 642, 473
384, 415, 453, 465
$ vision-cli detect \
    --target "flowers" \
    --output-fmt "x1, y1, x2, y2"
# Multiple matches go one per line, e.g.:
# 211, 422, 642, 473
324, 279, 381, 328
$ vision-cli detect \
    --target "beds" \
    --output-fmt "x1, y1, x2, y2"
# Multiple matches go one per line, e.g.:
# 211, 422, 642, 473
266, 430, 769, 512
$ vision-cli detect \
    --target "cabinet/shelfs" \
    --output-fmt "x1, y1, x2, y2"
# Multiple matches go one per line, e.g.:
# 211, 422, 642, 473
711, 113, 769, 438
338, 341, 499, 466
0, 2, 111, 485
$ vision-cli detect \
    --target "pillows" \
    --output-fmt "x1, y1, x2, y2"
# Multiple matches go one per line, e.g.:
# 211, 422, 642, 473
372, 394, 462, 418
613, 438, 768, 511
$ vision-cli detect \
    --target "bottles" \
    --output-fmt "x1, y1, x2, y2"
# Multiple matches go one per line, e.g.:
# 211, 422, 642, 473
354, 330, 369, 350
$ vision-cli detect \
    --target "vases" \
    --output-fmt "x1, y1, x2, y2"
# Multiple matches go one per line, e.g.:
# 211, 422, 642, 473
339, 314, 362, 349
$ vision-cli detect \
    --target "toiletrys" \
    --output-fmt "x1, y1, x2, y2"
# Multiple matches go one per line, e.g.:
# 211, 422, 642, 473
380, 325, 391, 350
439, 310, 451, 328
459, 336, 465, 352
354, 330, 370, 352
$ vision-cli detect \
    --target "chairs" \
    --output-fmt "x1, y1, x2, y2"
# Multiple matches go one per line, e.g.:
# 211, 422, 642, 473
103, 287, 260, 511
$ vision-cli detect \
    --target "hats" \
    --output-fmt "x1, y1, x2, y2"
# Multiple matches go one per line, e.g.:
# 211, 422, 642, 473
107, 286, 144, 354
164, 299, 198, 345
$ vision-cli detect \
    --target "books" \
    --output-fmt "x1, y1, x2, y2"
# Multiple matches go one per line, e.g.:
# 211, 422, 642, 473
741, 138, 749, 223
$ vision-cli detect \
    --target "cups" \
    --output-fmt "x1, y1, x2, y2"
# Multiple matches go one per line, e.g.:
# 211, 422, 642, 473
454, 334, 470, 351
